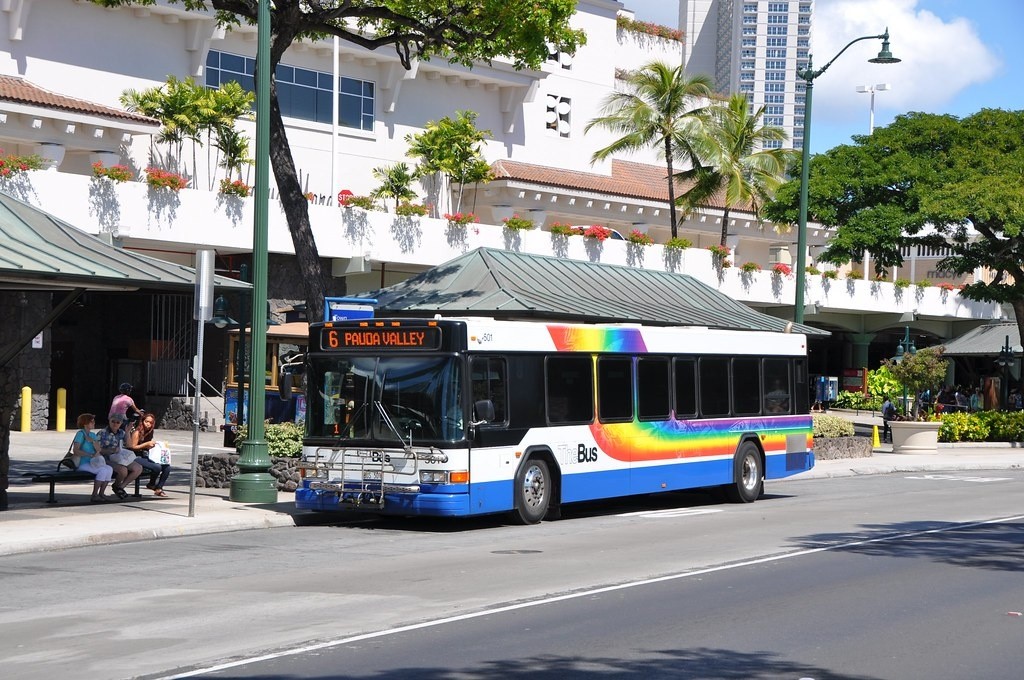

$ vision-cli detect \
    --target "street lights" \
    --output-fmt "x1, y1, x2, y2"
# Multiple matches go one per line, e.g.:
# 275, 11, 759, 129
792, 25, 904, 323
855, 81, 889, 282
895, 325, 917, 416
993, 334, 1016, 414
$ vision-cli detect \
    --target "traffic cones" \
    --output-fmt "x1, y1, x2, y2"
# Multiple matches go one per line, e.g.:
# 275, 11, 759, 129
870, 423, 882, 448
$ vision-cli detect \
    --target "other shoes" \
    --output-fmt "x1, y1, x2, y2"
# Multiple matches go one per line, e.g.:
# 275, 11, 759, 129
112, 484, 128, 499
99, 494, 110, 500
91, 495, 105, 502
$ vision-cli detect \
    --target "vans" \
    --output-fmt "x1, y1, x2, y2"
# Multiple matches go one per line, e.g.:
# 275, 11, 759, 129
569, 224, 630, 242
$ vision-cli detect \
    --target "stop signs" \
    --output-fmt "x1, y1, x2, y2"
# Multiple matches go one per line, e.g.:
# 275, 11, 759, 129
337, 189, 355, 205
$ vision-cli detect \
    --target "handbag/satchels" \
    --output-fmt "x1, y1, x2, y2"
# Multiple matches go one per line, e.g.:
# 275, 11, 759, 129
149, 441, 171, 465
62, 452, 81, 470
109, 440, 137, 466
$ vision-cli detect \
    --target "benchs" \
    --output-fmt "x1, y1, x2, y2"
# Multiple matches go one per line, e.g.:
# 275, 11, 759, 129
23, 458, 162, 503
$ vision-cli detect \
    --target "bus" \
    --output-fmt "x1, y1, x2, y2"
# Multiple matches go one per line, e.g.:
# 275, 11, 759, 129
279, 312, 815, 526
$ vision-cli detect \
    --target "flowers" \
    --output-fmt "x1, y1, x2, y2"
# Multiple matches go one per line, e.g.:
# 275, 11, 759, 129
0, 148, 53, 179
502, 213, 534, 232
583, 224, 613, 242
219, 178, 250, 198
704, 245, 732, 268
548, 221, 583, 236
90, 159, 132, 184
936, 282, 955, 290
821, 269, 840, 280
627, 229, 655, 247
616, 13, 687, 46
739, 262, 762, 273
303, 192, 314, 201
393, 203, 433, 217
958, 283, 966, 289
444, 211, 480, 235
770, 262, 791, 277
872, 274, 890, 282
143, 167, 189, 192
615, 67, 725, 103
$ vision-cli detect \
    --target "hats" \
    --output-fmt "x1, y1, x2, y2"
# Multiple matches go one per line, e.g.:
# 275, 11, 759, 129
110, 413, 123, 423
120, 383, 133, 389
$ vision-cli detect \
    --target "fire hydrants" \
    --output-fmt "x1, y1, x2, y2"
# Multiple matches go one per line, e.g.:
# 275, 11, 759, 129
935, 404, 944, 419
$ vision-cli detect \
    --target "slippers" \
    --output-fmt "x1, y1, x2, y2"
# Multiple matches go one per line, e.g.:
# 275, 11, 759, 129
154, 491, 168, 497
146, 484, 156, 490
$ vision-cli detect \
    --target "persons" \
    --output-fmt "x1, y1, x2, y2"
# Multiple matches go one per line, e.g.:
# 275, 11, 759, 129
767, 377, 789, 414
73, 414, 114, 503
108, 383, 144, 422
449, 393, 462, 428
882, 395, 898, 443
122, 408, 172, 497
919, 383, 985, 414
96, 413, 143, 499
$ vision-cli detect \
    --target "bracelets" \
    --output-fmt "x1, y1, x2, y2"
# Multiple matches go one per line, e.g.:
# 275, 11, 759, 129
90, 454, 93, 458
93, 440, 96, 443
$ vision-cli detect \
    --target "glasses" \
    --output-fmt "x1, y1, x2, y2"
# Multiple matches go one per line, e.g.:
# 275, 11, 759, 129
112, 421, 120, 425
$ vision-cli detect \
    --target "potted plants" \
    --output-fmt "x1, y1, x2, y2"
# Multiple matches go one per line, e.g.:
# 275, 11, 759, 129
883, 344, 951, 456
